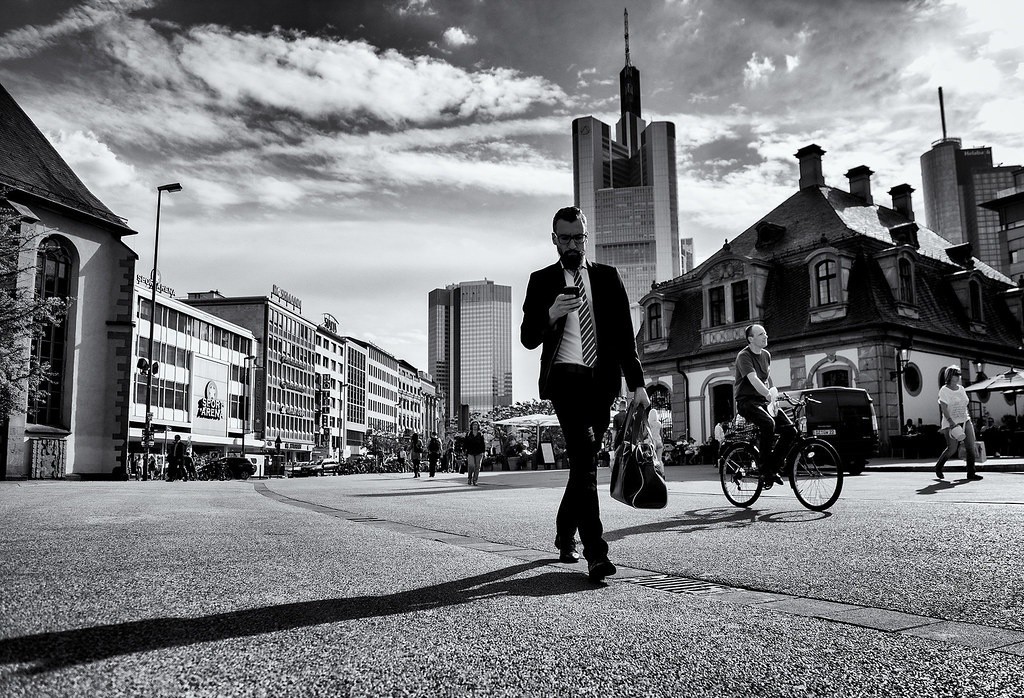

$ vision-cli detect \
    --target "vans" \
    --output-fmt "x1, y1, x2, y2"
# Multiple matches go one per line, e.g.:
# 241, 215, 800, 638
717, 385, 880, 476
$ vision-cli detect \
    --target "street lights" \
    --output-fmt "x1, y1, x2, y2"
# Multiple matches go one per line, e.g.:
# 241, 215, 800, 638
241, 354, 256, 456
142, 183, 184, 481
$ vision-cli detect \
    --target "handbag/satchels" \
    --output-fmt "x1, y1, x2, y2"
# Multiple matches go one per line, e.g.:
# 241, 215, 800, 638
959, 442, 987, 463
414, 442, 423, 453
609, 401, 668, 510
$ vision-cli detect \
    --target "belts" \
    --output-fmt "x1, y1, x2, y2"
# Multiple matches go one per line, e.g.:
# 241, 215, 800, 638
552, 367, 599, 379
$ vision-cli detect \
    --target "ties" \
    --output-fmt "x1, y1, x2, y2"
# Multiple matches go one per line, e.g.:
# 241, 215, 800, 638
572, 266, 598, 368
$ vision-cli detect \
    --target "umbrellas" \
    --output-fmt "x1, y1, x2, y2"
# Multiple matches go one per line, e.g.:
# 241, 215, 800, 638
492, 414, 560, 449
964, 365, 1024, 416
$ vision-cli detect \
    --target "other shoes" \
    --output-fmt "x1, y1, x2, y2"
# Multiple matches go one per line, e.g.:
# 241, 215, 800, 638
587, 558, 616, 582
473, 482, 478, 486
756, 463, 783, 485
468, 481, 472, 485
555, 536, 579, 562
413, 474, 417, 478
418, 473, 421, 478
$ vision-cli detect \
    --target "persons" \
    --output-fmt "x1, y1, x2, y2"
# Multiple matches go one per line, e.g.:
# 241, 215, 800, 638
648, 409, 665, 480
427, 432, 442, 477
733, 324, 798, 486
462, 421, 485, 485
934, 365, 983, 481
979, 417, 1000, 457
613, 400, 635, 451
400, 447, 407, 473
408, 433, 422, 478
137, 454, 162, 480
662, 418, 726, 468
520, 207, 652, 582
902, 419, 918, 459
166, 435, 187, 482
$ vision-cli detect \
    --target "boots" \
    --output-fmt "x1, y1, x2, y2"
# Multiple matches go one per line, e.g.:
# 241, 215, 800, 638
935, 453, 947, 479
967, 461, 983, 480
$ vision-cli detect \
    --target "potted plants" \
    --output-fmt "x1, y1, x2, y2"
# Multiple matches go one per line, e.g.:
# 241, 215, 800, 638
504, 442, 527, 471
557, 454, 562, 469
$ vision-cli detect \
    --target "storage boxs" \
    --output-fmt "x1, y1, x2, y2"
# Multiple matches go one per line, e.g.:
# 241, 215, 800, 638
545, 465, 556, 469
538, 465, 544, 470
522, 460, 533, 470
483, 462, 502, 471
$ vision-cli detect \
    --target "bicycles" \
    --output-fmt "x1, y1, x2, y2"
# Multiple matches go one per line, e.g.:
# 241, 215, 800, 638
715, 391, 844, 513
184, 462, 233, 482
341, 463, 402, 472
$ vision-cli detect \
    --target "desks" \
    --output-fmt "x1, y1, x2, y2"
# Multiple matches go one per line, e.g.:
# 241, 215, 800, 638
888, 434, 924, 459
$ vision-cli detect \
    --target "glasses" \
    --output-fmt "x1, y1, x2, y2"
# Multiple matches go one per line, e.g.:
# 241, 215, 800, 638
554, 230, 587, 244
952, 373, 961, 378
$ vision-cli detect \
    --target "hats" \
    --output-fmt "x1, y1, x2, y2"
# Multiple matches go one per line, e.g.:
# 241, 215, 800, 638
949, 425, 966, 442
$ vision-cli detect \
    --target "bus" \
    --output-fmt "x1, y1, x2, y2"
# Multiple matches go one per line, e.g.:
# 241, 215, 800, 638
367, 452, 392, 462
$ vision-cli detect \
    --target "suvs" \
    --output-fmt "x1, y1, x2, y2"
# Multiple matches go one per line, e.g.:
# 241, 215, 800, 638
303, 458, 339, 476
197, 457, 257, 480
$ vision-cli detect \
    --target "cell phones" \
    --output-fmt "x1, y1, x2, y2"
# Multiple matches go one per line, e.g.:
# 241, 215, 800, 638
564, 286, 579, 301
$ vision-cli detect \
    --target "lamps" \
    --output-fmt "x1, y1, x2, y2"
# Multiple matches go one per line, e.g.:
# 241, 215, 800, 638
972, 355, 987, 378
890, 336, 913, 383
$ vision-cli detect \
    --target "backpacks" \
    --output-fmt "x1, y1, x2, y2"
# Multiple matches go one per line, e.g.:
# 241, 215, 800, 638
429, 436, 440, 453
